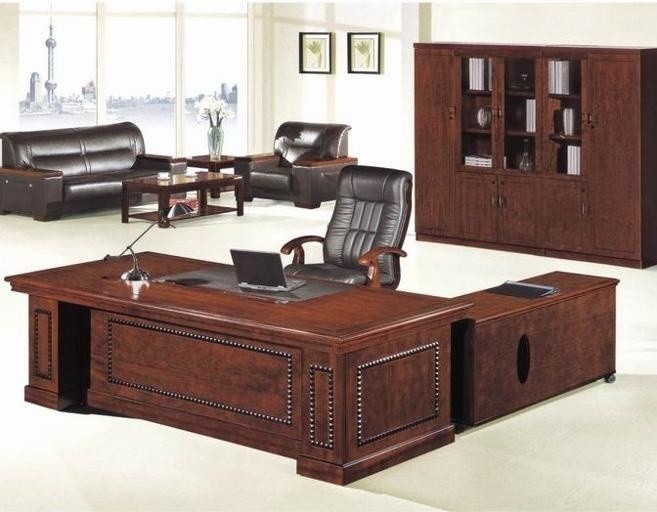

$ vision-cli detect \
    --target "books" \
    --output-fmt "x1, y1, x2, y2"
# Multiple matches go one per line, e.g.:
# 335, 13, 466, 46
465, 58, 581, 176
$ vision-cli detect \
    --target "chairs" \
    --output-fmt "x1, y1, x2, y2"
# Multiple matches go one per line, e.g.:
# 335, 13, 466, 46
280, 164, 413, 291
233, 121, 359, 210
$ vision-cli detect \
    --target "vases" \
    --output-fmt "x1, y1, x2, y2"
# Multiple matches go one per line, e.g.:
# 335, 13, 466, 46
206, 125, 225, 163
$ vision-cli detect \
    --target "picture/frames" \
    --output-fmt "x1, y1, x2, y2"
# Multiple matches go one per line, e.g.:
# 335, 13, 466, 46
346, 32, 381, 76
298, 31, 332, 75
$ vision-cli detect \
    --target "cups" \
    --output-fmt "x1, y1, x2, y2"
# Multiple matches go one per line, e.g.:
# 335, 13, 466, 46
186, 178, 196, 182
158, 181, 168, 186
186, 167, 194, 175
158, 172, 168, 178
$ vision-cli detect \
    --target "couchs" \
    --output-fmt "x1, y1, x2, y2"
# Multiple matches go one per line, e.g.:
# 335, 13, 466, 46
0, 120, 188, 222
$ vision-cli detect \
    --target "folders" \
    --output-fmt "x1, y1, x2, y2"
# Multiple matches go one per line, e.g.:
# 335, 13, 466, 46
485, 281, 555, 299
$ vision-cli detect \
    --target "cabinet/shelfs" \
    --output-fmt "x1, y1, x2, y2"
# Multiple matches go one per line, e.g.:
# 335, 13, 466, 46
412, 42, 547, 259
539, 45, 657, 270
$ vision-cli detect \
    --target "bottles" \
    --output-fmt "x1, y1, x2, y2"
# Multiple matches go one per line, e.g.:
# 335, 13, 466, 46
518, 139, 533, 172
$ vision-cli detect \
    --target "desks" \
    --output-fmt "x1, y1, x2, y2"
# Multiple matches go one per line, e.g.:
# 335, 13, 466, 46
189, 152, 238, 200
450, 268, 621, 431
2, 251, 477, 488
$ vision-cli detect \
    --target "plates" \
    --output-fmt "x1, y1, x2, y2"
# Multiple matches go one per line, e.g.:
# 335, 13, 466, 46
156, 177, 170, 181
183, 174, 197, 177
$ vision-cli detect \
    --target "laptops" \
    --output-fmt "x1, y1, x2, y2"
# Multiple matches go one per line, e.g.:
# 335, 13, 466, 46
230, 248, 307, 292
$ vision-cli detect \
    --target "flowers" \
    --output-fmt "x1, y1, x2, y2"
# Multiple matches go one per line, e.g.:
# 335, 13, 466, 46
196, 98, 235, 129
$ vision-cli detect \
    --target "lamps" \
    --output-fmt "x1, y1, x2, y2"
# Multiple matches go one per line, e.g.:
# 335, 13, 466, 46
98, 200, 198, 285
